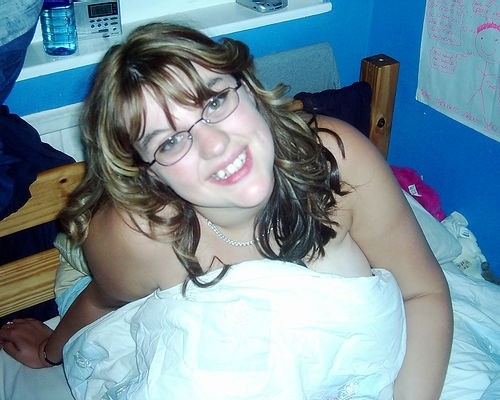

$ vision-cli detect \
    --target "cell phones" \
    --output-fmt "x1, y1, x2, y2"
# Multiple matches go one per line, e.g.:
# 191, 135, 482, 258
236, 0, 288, 13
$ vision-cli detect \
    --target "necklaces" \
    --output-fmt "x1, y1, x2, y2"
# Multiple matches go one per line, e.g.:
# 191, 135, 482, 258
206, 220, 275, 247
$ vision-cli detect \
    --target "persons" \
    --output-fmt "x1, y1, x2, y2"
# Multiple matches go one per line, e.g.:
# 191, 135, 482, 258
0, 22, 453, 400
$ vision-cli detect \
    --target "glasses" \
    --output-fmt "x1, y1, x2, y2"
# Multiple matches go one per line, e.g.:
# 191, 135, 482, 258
142, 81, 242, 168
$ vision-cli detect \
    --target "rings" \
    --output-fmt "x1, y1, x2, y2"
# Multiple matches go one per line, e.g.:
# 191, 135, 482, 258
3, 321, 16, 329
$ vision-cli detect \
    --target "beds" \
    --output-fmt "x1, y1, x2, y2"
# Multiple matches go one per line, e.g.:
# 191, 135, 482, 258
0, 53, 499, 398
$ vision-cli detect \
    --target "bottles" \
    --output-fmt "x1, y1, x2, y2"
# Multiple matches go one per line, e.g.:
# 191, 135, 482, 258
39, 0, 79, 56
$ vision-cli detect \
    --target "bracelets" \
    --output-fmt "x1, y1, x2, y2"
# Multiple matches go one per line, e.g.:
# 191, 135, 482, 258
43, 343, 63, 365
